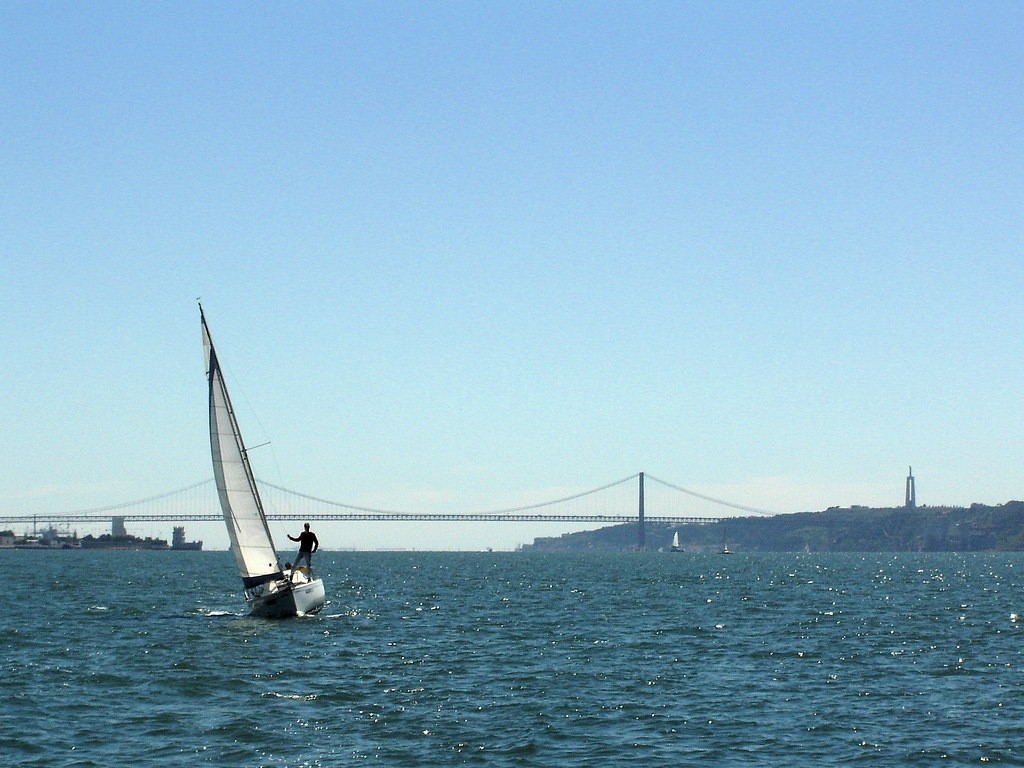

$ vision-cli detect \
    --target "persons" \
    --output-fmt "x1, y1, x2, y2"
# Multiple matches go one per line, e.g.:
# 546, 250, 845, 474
287, 523, 318, 583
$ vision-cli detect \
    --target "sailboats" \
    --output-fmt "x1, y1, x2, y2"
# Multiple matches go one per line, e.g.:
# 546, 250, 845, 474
723, 543, 736, 555
197, 302, 327, 619
671, 531, 685, 553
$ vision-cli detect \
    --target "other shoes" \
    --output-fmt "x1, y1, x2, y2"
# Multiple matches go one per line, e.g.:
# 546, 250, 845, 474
308, 579, 315, 583
